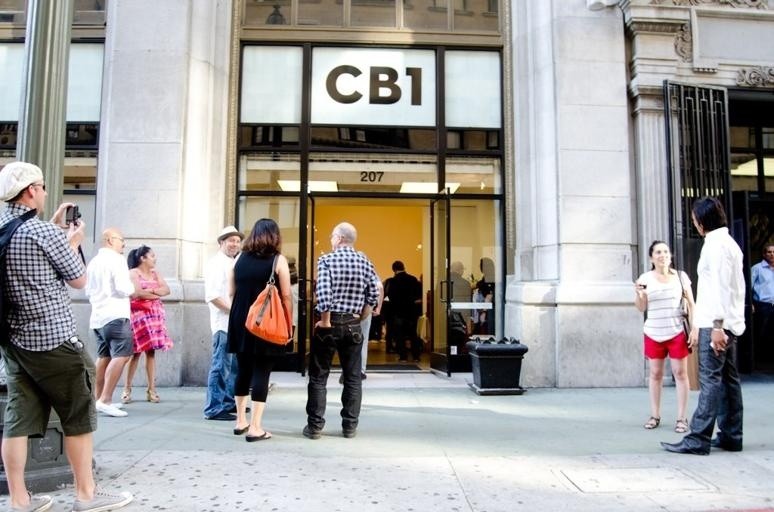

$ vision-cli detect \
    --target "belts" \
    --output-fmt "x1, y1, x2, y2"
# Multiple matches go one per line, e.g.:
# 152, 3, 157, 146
331, 312, 354, 316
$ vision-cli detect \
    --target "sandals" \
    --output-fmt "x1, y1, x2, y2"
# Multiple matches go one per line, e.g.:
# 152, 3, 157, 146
643, 416, 660, 429
675, 418, 688, 433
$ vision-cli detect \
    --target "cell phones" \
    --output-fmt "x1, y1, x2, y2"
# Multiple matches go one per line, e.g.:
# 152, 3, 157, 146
710, 334, 729, 348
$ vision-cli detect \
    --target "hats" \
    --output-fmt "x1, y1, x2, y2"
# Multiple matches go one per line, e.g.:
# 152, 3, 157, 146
0, 162, 43, 202
217, 225, 245, 245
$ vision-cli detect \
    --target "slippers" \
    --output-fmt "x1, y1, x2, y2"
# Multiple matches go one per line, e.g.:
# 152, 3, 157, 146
246, 431, 271, 442
234, 423, 250, 435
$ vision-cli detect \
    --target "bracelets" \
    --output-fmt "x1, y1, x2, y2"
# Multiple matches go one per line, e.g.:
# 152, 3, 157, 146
151, 288, 155, 294
711, 327, 723, 333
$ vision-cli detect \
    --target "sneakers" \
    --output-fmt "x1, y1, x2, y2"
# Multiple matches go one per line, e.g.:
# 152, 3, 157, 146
95, 400, 128, 417
113, 403, 123, 409
9, 492, 53, 512
72, 483, 134, 512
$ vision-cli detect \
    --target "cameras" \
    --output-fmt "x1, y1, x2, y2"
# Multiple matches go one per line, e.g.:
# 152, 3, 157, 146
66, 205, 81, 225
640, 285, 647, 289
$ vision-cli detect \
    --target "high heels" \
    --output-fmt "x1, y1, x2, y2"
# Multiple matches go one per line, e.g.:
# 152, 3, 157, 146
146, 389, 160, 403
121, 387, 131, 404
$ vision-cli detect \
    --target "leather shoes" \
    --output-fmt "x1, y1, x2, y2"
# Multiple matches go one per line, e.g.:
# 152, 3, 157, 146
207, 410, 236, 420
227, 404, 250, 413
661, 440, 696, 454
343, 428, 355, 438
303, 425, 321, 439
710, 440, 721, 447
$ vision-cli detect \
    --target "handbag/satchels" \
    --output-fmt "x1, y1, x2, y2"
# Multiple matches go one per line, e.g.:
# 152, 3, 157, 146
677, 270, 689, 319
130, 298, 153, 310
244, 253, 293, 346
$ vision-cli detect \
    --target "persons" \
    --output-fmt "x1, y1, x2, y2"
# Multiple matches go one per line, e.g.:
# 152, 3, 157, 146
120, 246, 174, 402
0, 161, 134, 512
225, 218, 293, 441
303, 221, 383, 439
339, 276, 385, 383
750, 244, 774, 376
659, 195, 746, 454
632, 239, 696, 432
384, 258, 496, 363
203, 226, 250, 422
83, 227, 139, 418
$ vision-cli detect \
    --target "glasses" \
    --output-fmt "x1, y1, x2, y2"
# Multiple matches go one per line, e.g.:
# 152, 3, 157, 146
32, 184, 46, 191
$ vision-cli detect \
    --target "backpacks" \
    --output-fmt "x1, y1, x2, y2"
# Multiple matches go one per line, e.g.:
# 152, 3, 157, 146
0, 207, 37, 347
